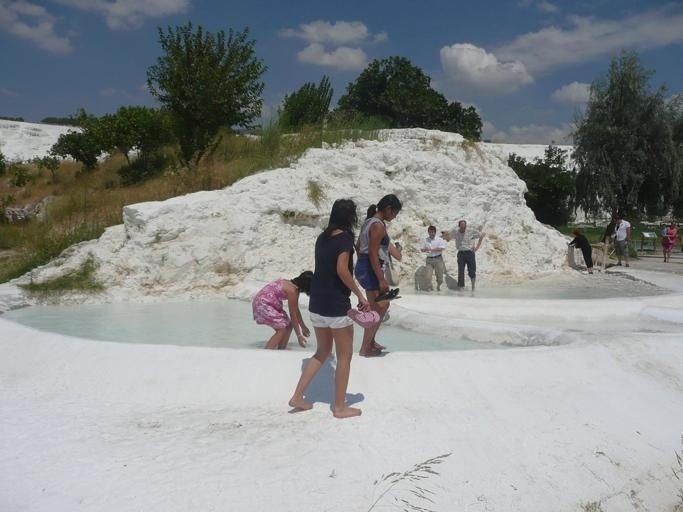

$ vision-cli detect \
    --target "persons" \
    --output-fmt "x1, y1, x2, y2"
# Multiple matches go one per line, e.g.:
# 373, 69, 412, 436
252, 270, 314, 349
661, 224, 673, 243
661, 223, 676, 263
613, 214, 631, 267
568, 228, 593, 274
288, 199, 370, 418
355, 194, 401, 357
455, 220, 483, 297
421, 225, 445, 292
388, 242, 403, 262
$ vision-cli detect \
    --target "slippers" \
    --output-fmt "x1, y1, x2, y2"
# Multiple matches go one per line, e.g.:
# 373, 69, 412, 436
375, 288, 398, 302
347, 308, 380, 327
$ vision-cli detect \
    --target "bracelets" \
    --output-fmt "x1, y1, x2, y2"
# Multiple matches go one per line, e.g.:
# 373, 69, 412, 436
298, 335, 302, 337
